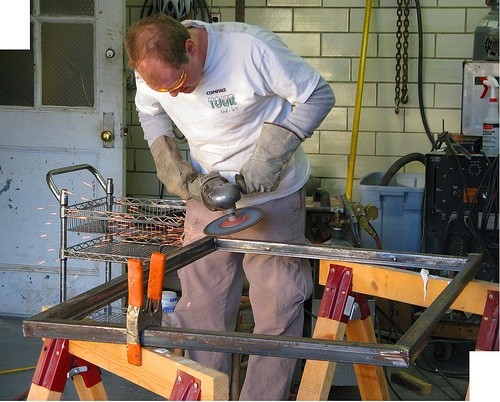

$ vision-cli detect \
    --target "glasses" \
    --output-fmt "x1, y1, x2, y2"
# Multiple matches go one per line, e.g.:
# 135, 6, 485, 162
156, 57, 187, 92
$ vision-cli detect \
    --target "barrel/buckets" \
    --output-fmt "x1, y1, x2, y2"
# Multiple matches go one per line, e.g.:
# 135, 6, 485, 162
162, 290, 178, 313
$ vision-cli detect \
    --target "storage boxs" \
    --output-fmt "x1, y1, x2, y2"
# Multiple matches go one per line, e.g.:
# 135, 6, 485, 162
359, 172, 425, 253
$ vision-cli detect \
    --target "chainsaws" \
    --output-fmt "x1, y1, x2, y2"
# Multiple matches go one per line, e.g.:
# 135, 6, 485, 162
186, 170, 266, 237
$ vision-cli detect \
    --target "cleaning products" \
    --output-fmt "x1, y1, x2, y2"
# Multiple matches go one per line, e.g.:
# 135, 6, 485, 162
479, 76, 499, 157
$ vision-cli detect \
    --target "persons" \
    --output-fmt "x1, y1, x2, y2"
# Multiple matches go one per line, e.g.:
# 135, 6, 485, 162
124, 14, 336, 401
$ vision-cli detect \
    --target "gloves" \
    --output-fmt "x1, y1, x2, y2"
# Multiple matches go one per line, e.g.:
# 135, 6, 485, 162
150, 135, 200, 200
239, 122, 301, 194
